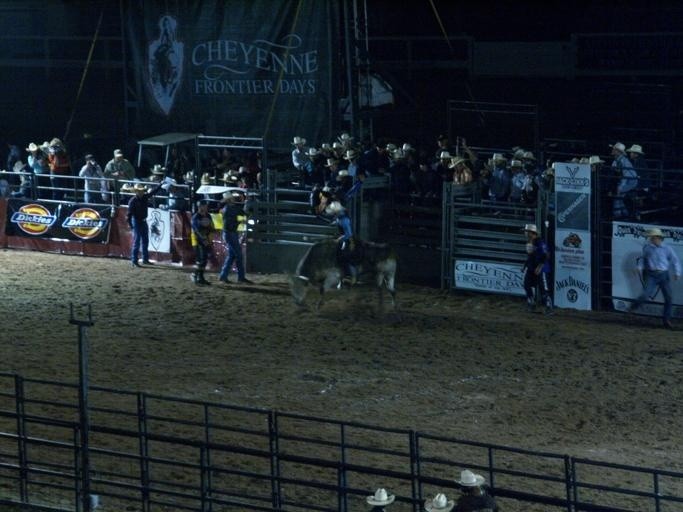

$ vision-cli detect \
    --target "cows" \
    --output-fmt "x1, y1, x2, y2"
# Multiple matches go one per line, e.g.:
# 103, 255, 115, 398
287, 240, 412, 308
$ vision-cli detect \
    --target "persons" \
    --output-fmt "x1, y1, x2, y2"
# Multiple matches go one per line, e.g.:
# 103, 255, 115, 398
422, 493, 456, 512
0, 130, 659, 227
365, 488, 396, 512
623, 229, 680, 331
452, 469, 499, 512
517, 219, 555, 318
123, 182, 163, 267
194, 190, 256, 286
325, 197, 362, 283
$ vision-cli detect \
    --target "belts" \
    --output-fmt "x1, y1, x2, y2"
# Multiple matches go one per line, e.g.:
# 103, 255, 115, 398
647, 270, 664, 273
133, 218, 146, 222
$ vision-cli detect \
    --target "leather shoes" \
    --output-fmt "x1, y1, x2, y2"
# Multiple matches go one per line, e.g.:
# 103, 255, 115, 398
131, 263, 143, 268
190, 270, 253, 285
626, 307, 635, 312
662, 320, 676, 329
142, 260, 154, 265
351, 275, 358, 285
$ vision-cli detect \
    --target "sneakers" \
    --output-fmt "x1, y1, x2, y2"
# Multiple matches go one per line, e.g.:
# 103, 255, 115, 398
543, 308, 556, 315
526, 307, 537, 312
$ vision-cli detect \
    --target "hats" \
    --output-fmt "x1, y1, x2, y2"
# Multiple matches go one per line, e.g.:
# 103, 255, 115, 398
129, 183, 146, 191
183, 166, 249, 187
625, 144, 644, 156
436, 147, 537, 170
520, 223, 541, 235
26, 137, 64, 152
647, 228, 666, 238
570, 155, 606, 166
150, 164, 168, 175
435, 133, 450, 141
289, 133, 416, 181
608, 142, 627, 154
326, 202, 346, 213
196, 200, 209, 206
220, 192, 239, 202
85, 154, 94, 159
113, 149, 125, 157
365, 469, 485, 511
